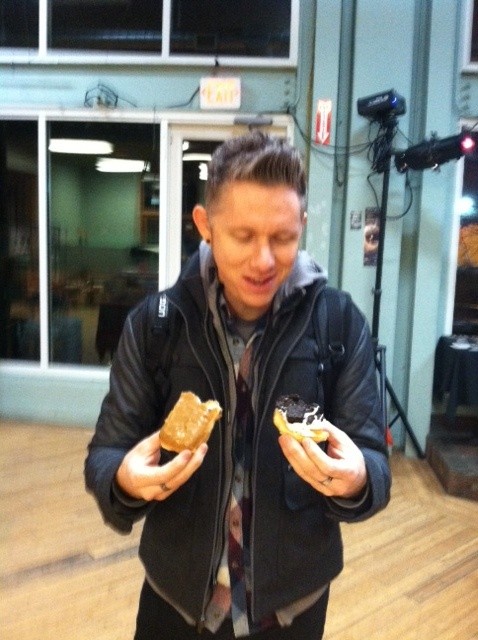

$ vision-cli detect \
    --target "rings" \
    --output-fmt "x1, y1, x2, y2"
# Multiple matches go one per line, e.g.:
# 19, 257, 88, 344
159, 483, 171, 493
317, 475, 333, 487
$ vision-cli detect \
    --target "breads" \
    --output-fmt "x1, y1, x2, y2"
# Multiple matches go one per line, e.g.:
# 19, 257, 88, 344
156, 390, 222, 453
272, 393, 328, 447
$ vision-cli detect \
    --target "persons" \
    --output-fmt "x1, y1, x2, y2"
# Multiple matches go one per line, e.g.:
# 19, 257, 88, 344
84, 130, 392, 639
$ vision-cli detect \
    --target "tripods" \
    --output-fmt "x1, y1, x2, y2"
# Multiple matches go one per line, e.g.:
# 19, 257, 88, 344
370, 121, 427, 460
